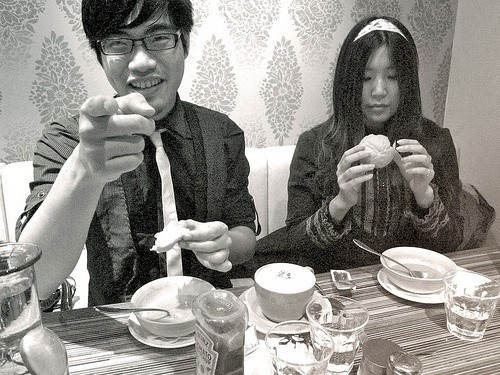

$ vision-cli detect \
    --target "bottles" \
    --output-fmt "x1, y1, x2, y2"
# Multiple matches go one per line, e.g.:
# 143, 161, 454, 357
356, 338, 402, 375
195, 291, 248, 375
386, 351, 424, 375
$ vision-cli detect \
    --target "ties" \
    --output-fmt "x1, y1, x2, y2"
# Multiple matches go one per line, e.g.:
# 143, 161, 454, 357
148, 127, 184, 276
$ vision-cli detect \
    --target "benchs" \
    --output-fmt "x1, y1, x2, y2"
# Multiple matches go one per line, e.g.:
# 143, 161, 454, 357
0, 146, 298, 313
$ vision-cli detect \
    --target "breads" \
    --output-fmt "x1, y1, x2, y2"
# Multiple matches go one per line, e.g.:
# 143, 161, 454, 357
149, 220, 190, 253
358, 134, 396, 168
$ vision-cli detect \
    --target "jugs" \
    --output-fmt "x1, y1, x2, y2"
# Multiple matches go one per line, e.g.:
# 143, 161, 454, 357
0, 240, 71, 375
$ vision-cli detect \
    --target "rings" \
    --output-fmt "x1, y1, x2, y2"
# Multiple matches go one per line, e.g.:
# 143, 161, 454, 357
426, 169, 430, 177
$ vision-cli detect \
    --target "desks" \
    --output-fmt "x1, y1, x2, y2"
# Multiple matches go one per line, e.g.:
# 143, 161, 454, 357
0, 245, 500, 375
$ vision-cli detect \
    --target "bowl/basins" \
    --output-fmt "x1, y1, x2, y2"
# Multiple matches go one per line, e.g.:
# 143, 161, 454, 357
131, 276, 217, 337
379, 246, 457, 293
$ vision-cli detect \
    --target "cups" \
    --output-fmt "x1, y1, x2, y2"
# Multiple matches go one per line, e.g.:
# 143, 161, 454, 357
443, 270, 500, 342
254, 262, 316, 323
264, 320, 334, 375
306, 295, 369, 375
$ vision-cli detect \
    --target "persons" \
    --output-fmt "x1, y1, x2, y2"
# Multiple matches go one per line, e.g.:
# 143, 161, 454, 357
285, 16, 464, 274
16, 0, 262, 308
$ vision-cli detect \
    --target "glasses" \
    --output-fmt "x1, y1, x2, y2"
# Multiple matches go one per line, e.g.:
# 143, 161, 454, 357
97, 27, 183, 55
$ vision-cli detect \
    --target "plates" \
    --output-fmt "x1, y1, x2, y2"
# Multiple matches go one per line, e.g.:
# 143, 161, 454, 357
128, 311, 195, 348
377, 267, 468, 303
240, 288, 332, 334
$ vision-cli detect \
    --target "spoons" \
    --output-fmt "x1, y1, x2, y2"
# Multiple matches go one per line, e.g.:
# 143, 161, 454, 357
314, 283, 345, 309
352, 238, 429, 278
